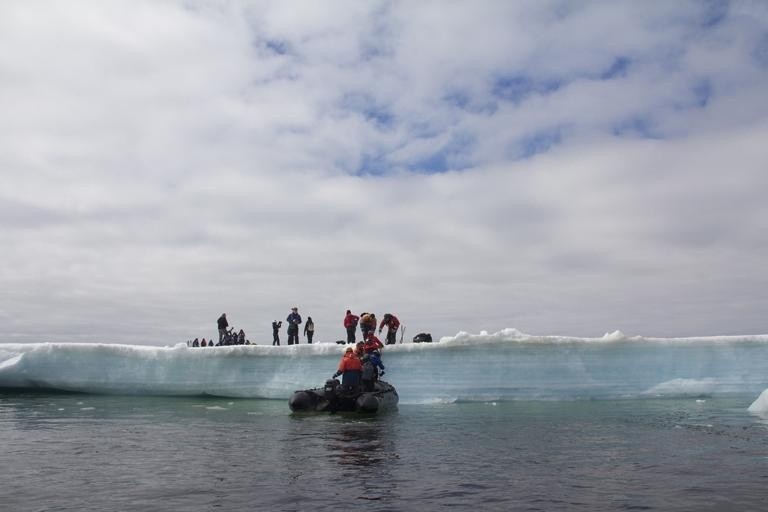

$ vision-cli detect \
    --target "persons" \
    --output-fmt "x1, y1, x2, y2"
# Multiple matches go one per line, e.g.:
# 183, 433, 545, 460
286, 306, 302, 345
272, 320, 283, 346
378, 313, 400, 344
360, 312, 378, 340
188, 312, 257, 348
303, 316, 315, 344
344, 309, 359, 344
334, 331, 386, 395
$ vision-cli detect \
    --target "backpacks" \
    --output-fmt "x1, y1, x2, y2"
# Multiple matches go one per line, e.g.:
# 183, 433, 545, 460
306, 320, 314, 332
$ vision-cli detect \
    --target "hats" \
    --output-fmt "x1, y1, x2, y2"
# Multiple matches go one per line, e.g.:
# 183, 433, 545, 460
291, 307, 297, 310
272, 320, 277, 323
345, 347, 353, 352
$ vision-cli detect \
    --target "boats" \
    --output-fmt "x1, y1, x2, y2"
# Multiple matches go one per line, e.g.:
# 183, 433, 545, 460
288, 380, 398, 413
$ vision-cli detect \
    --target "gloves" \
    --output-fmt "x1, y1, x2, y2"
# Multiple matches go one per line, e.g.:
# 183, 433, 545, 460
332, 374, 338, 378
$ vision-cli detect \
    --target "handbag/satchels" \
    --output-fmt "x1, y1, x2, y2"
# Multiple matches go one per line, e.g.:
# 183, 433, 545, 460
413, 333, 433, 342
